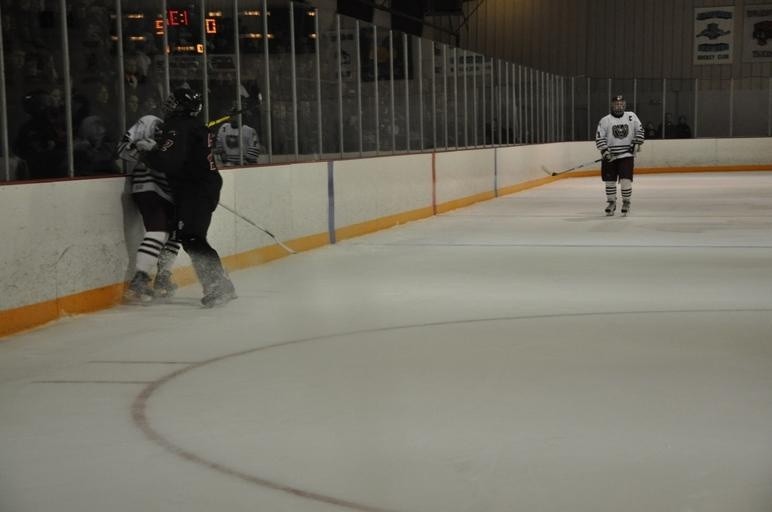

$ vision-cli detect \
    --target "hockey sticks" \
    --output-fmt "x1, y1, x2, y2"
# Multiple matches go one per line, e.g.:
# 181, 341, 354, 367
541, 151, 629, 176
218, 199, 297, 255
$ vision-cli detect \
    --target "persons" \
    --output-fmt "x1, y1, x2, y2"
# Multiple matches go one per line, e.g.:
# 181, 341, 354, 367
644, 121, 658, 139
139, 87, 234, 305
115, 115, 182, 296
490, 118, 506, 144
1, 4, 476, 183
656, 113, 673, 139
670, 115, 692, 138
595, 94, 646, 213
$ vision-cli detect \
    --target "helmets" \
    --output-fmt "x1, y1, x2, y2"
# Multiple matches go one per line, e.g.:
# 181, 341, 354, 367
169, 78, 204, 117
611, 95, 625, 102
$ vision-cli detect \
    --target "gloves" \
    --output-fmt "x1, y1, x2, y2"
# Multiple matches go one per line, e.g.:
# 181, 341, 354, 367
131, 136, 158, 153
600, 147, 612, 162
630, 139, 644, 153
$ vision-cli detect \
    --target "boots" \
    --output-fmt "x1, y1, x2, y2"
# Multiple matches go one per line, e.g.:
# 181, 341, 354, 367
620, 200, 631, 213
152, 269, 180, 292
128, 269, 158, 296
604, 200, 617, 213
199, 270, 237, 305
